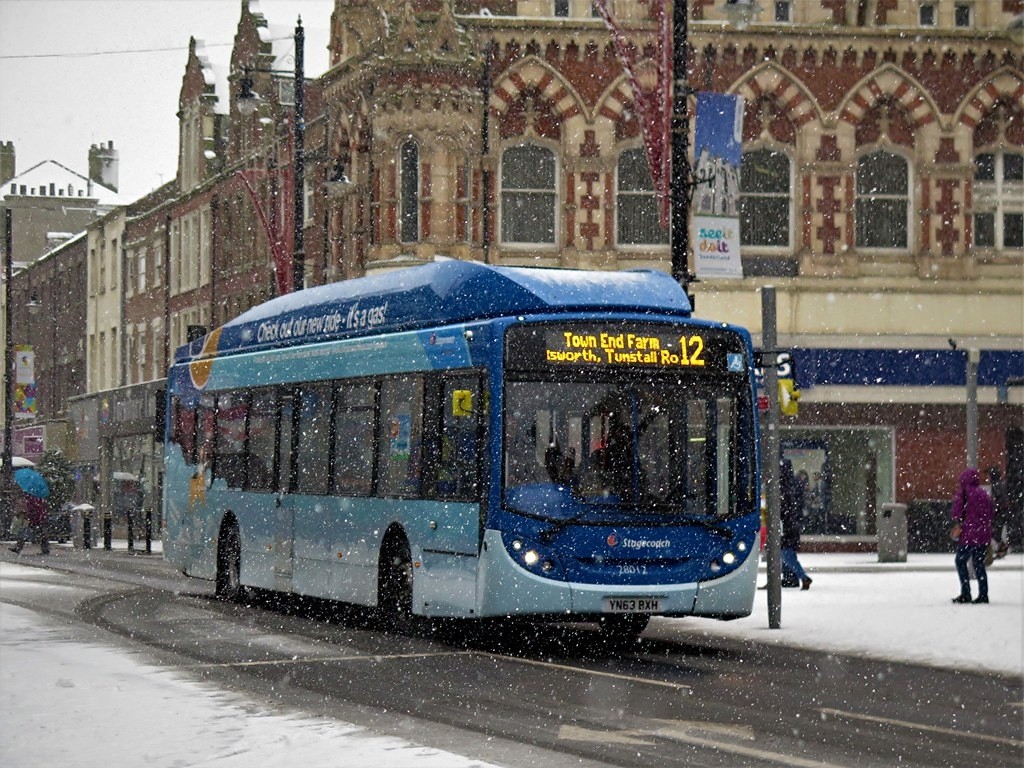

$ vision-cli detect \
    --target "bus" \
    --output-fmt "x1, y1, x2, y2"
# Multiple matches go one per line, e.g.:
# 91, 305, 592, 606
163, 251, 796, 652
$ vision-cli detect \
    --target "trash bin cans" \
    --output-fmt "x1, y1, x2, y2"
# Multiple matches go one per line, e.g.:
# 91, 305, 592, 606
878, 504, 908, 562
73, 505, 93, 551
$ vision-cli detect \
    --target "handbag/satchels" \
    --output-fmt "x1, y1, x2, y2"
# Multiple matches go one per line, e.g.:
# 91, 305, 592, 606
6, 514, 31, 542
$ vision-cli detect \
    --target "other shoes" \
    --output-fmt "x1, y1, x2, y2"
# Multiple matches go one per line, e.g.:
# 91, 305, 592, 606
952, 592, 973, 604
972, 594, 989, 604
801, 575, 812, 591
6, 547, 21, 555
757, 582, 768, 591
779, 574, 799, 588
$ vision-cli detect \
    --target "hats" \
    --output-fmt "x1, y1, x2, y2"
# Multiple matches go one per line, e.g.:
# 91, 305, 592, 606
778, 458, 794, 477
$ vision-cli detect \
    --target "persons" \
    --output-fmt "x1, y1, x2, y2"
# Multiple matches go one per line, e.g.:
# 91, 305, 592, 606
589, 425, 648, 493
951, 469, 997, 604
757, 459, 825, 590
8, 487, 50, 556
983, 460, 1024, 554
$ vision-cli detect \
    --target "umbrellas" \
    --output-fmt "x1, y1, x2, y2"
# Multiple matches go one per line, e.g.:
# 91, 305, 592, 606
0, 457, 35, 467
15, 468, 50, 498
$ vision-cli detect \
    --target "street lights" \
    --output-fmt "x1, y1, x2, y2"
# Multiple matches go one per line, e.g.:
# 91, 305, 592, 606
24, 291, 58, 419
235, 66, 305, 290
960, 346, 982, 467
